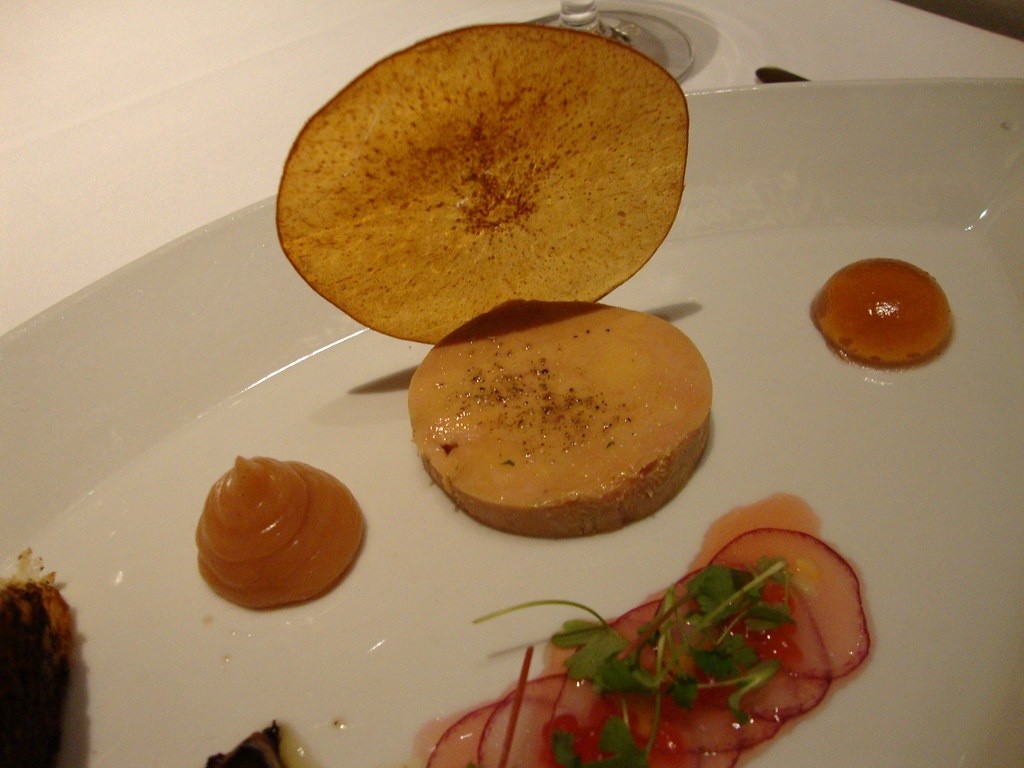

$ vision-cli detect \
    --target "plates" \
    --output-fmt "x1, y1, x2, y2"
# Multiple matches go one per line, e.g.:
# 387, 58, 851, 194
3, 81, 1022, 767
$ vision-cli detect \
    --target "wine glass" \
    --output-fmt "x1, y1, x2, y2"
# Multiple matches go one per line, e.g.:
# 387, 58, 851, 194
522, 0, 694, 88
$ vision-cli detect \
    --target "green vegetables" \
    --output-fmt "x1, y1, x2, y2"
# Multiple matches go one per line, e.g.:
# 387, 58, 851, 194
475, 555, 798, 767
